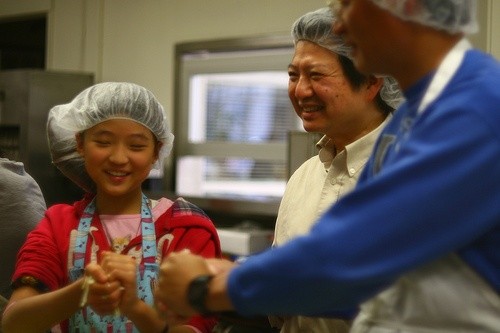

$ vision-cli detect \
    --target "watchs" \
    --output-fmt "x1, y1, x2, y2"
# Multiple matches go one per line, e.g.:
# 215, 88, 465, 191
186, 274, 211, 317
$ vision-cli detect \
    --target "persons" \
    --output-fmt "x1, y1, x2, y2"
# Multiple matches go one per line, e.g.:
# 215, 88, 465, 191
269, 7, 404, 333
154, 0, 500, 333
0, 158, 47, 301
0, 82, 221, 332
